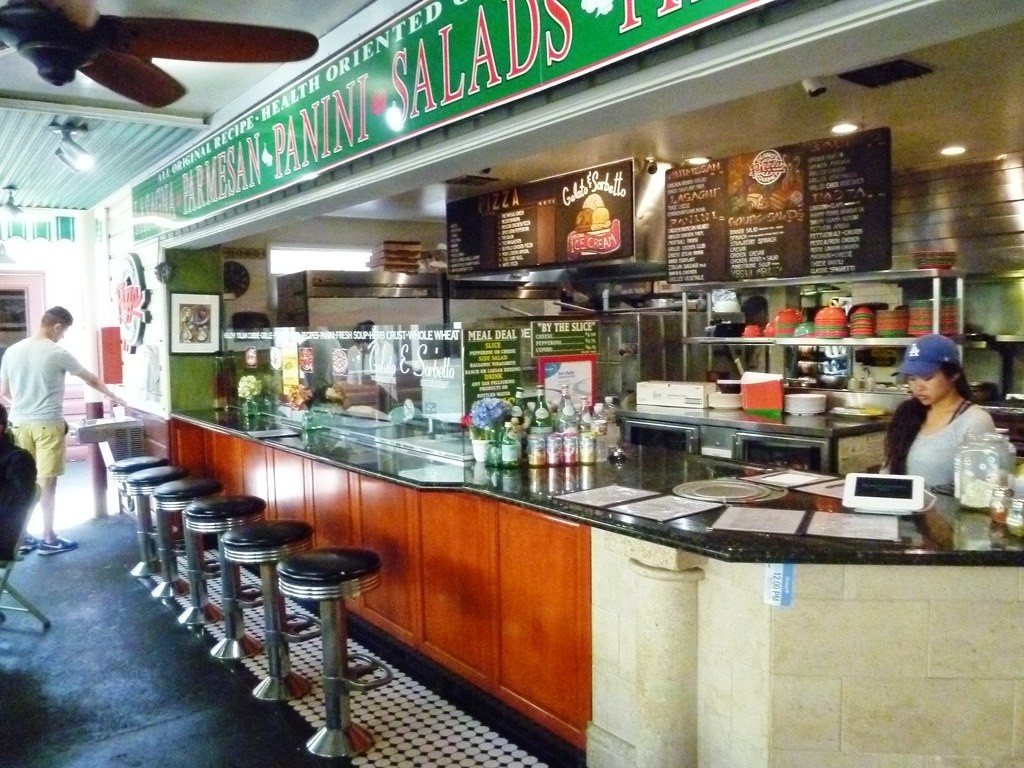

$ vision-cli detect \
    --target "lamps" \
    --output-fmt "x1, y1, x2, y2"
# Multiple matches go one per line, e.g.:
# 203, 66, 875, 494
3, 186, 24, 218
49, 122, 96, 174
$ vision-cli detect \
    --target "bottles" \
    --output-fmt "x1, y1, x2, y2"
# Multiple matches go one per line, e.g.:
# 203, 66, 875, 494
989, 487, 1014, 525
848, 362, 874, 392
487, 383, 626, 468
1005, 499, 1024, 540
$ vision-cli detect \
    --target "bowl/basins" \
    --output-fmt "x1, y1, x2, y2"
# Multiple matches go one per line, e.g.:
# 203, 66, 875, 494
774, 308, 815, 337
849, 306, 875, 338
715, 323, 746, 337
800, 290, 849, 307
814, 307, 848, 338
968, 381, 993, 401
716, 379, 741, 394
819, 374, 847, 389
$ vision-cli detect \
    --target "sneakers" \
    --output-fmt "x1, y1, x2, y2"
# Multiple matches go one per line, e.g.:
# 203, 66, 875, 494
20, 535, 43, 551
38, 536, 79, 555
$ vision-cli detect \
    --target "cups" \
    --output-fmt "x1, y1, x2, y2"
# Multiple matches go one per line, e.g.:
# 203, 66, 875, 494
744, 325, 759, 334
765, 324, 774, 332
113, 403, 124, 422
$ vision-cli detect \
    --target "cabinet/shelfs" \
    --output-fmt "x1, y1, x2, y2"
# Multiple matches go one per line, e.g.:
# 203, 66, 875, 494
671, 268, 964, 382
964, 334, 1024, 415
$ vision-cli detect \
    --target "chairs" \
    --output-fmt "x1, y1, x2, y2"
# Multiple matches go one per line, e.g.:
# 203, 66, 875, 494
0, 484, 52, 629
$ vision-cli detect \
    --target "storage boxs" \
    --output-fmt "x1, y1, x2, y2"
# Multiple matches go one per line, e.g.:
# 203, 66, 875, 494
636, 379, 717, 409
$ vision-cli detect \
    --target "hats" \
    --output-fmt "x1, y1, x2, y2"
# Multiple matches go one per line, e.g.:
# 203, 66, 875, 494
892, 334, 959, 379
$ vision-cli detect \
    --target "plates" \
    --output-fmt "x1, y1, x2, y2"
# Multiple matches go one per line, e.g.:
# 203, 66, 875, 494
875, 297, 984, 338
741, 332, 761, 337
828, 409, 884, 419
763, 332, 774, 336
913, 250, 956, 269
708, 374, 827, 420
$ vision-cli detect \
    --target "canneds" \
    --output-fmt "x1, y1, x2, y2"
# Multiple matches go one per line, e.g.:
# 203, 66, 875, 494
526, 432, 596, 466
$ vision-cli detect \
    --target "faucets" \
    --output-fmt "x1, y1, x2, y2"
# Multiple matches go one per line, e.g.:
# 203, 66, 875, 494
861, 367, 871, 378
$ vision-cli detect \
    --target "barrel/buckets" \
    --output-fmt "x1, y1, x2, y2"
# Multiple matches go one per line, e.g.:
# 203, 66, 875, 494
954, 429, 1016, 513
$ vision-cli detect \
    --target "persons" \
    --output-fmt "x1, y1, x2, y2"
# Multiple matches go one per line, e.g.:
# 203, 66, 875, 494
877, 334, 997, 495
0, 404, 37, 551
0, 305, 128, 554
710, 295, 768, 382
347, 320, 395, 394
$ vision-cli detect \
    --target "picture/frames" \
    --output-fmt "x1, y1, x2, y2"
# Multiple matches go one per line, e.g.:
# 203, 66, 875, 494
0, 291, 26, 331
169, 290, 224, 356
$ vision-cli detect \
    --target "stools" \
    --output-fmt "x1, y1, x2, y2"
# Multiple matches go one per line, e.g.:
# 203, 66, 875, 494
183, 496, 266, 659
127, 465, 191, 600
221, 519, 324, 702
152, 477, 225, 625
108, 455, 170, 579
275, 546, 392, 759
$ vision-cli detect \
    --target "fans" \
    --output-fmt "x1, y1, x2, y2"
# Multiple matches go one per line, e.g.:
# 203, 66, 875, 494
0, 0, 319, 107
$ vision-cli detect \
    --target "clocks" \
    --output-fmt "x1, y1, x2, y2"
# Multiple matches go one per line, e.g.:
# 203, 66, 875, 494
224, 262, 251, 298
